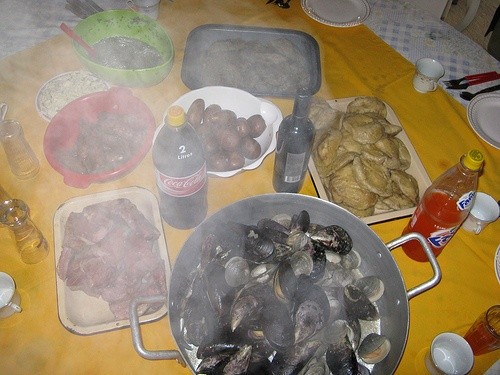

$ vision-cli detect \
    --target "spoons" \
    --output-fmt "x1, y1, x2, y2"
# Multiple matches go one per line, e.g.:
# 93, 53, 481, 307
459, 85, 500, 101
278, 0, 291, 9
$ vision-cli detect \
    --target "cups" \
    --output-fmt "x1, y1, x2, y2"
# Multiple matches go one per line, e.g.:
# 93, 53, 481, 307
0, 272, 22, 317
412, 57, 445, 94
425, 332, 474, 375
136, 0, 160, 20
462, 192, 500, 236
462, 305, 500, 356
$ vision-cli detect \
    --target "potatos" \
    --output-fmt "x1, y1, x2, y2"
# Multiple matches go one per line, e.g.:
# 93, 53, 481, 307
187, 98, 266, 172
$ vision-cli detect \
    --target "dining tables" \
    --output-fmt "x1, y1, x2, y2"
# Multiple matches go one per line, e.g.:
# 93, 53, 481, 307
0, 0, 500, 375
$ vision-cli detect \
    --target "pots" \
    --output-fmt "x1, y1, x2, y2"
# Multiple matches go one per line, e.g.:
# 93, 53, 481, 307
128, 193, 441, 375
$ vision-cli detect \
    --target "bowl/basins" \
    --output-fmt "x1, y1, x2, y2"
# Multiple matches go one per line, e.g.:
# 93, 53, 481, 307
42, 87, 155, 189
180, 24, 320, 99
152, 86, 284, 178
71, 10, 174, 87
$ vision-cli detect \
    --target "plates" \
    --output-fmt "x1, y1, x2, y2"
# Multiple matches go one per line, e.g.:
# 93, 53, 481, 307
35, 70, 111, 122
307, 98, 432, 224
301, 0, 370, 29
467, 92, 500, 151
493, 245, 500, 284
53, 186, 171, 335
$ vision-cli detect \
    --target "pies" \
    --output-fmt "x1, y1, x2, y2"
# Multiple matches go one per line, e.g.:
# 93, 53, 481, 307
305, 95, 419, 217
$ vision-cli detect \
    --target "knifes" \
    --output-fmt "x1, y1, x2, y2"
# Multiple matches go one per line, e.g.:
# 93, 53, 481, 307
446, 74, 500, 90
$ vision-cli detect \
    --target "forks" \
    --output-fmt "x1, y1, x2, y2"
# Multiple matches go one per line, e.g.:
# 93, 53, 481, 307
442, 71, 497, 87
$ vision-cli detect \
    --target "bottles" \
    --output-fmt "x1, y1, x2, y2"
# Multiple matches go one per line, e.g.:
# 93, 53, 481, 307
400, 149, 483, 263
0, 199, 50, 264
0, 120, 40, 178
152, 105, 208, 230
272, 87, 316, 193
